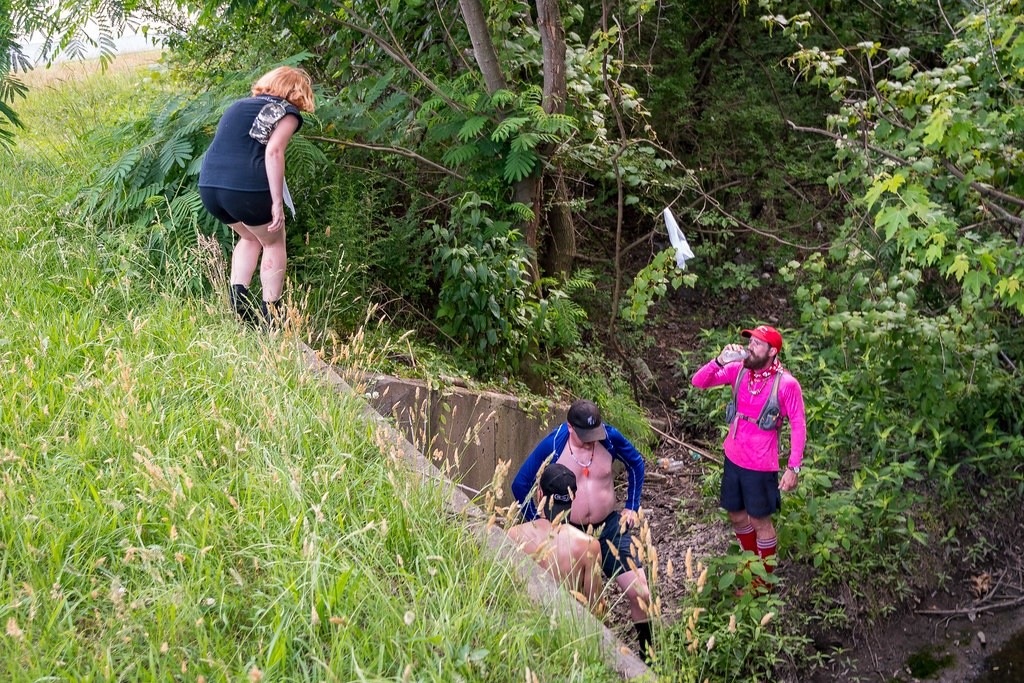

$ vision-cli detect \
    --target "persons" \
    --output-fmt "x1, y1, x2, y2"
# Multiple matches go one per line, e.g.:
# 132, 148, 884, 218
198, 67, 314, 335
691, 323, 806, 598
508, 461, 610, 630
512, 399, 655, 668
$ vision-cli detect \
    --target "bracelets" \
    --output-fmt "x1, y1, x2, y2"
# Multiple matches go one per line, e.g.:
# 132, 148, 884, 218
715, 357, 723, 367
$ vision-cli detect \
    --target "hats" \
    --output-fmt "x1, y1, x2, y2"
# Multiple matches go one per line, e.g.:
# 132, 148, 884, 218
540, 464, 577, 524
567, 400, 607, 442
740, 327, 784, 357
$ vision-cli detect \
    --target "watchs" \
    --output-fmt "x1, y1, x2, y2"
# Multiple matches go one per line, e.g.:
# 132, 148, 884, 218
789, 466, 802, 473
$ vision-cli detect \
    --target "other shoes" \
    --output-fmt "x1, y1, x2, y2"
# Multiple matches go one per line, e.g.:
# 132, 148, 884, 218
263, 300, 280, 328
230, 284, 258, 325
736, 577, 775, 597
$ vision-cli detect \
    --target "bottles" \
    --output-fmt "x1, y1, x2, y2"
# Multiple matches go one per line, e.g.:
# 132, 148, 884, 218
656, 458, 684, 471
722, 349, 752, 364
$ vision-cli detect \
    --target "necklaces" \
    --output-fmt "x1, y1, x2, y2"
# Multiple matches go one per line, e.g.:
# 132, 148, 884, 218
748, 379, 767, 395
568, 438, 595, 478
